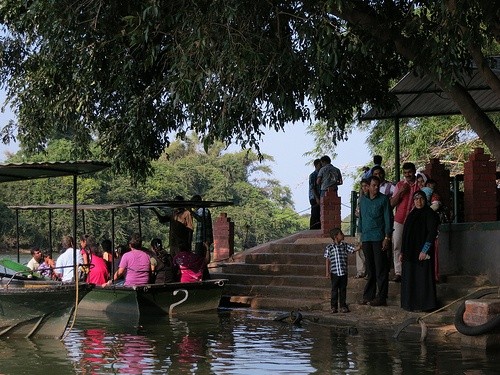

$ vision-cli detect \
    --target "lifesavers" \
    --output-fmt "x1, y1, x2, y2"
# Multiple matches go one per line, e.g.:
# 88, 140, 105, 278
454, 287, 500, 336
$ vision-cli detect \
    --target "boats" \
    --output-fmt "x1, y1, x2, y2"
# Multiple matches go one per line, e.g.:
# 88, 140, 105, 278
0, 160, 236, 334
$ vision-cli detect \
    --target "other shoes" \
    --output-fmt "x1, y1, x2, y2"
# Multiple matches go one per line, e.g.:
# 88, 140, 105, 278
341, 307, 349, 313
331, 309, 338, 313
391, 274, 401, 282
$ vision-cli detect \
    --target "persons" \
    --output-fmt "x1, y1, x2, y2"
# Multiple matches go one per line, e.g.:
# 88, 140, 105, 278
357, 176, 392, 307
28, 233, 203, 287
355, 155, 441, 283
151, 196, 194, 255
316, 155, 342, 198
323, 228, 361, 313
399, 190, 439, 313
309, 159, 321, 230
189, 195, 213, 248
